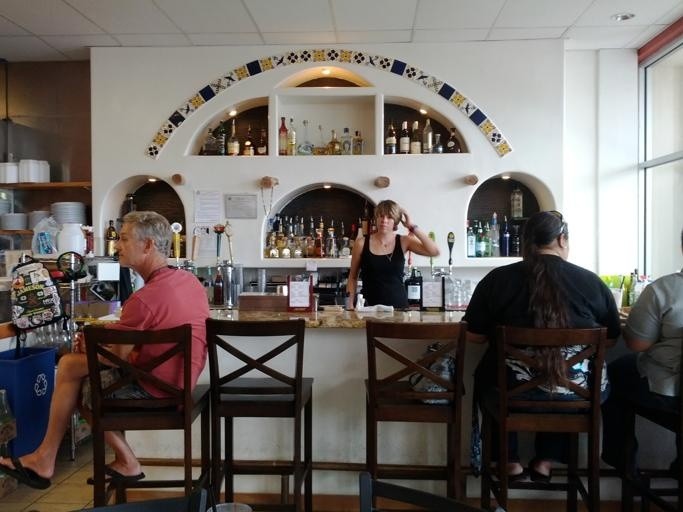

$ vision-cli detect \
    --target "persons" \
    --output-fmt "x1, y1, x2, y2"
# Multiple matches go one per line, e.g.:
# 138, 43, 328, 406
454, 211, 622, 479
624, 230, 683, 471
0, 210, 209, 488
346, 200, 439, 311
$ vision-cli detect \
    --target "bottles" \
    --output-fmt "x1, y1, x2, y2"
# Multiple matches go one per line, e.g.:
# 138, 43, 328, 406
226, 118, 240, 155
280, 238, 291, 258
349, 224, 357, 254
278, 117, 288, 154
483, 221, 490, 255
369, 208, 378, 234
422, 118, 432, 154
297, 121, 313, 155
308, 216, 315, 234
268, 219, 272, 231
634, 270, 641, 301
476, 221, 484, 257
339, 128, 353, 154
385, 119, 397, 153
315, 230, 325, 257
204, 266, 214, 301
327, 219, 335, 235
406, 267, 422, 307
266, 237, 279, 257
284, 216, 288, 230
467, 227, 476, 257
278, 218, 282, 231
313, 126, 328, 154
217, 119, 227, 154
294, 217, 301, 235
287, 218, 296, 234
300, 218, 304, 235
104, 221, 119, 258
292, 240, 303, 258
287, 118, 296, 154
327, 131, 339, 154
490, 213, 500, 257
500, 217, 510, 256
445, 127, 461, 153
202, 129, 214, 155
339, 237, 350, 257
243, 119, 254, 155
398, 121, 411, 153
338, 223, 346, 238
272, 214, 280, 231
319, 217, 325, 229
627, 273, 636, 305
466, 218, 470, 231
120, 194, 138, 214
433, 134, 443, 152
352, 131, 364, 154
410, 119, 421, 154
36, 316, 85, 368
362, 198, 369, 236
325, 228, 336, 257
215, 267, 223, 305
510, 182, 524, 221
256, 114, 268, 155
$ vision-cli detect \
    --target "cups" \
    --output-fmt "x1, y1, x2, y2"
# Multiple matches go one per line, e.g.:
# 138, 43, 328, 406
607, 287, 626, 315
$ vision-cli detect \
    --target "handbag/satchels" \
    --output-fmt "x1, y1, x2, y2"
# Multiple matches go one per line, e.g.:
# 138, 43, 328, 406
408, 344, 456, 404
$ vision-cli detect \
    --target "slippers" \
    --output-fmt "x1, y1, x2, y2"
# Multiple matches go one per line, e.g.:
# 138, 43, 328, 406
0, 456, 50, 490
497, 461, 526, 481
529, 457, 553, 482
87, 464, 146, 484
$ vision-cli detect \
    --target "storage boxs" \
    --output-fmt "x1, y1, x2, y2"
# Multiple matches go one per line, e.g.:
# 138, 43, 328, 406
67, 303, 112, 317
238, 293, 318, 320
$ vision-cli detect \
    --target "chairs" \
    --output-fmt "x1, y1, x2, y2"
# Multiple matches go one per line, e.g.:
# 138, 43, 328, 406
620, 395, 681, 511
364, 318, 467, 512
205, 317, 314, 512
480, 319, 608, 511
84, 324, 209, 512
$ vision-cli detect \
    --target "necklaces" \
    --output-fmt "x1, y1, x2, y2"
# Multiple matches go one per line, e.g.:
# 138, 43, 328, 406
373, 232, 397, 262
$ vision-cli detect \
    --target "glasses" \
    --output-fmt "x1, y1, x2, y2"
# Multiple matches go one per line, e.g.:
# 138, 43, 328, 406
522, 210, 567, 237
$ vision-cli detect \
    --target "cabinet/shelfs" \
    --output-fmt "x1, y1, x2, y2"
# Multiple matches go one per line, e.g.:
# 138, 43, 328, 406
0, 181, 91, 278
0, 286, 83, 504
96, 310, 624, 498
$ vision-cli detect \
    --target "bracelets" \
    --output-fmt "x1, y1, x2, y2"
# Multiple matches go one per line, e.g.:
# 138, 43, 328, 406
409, 225, 417, 232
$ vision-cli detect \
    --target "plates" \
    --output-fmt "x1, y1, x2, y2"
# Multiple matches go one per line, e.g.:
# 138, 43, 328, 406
0, 213, 26, 230
27, 210, 48, 230
0, 162, 19, 184
17, 159, 50, 182
49, 202, 86, 226
0, 192, 11, 216
